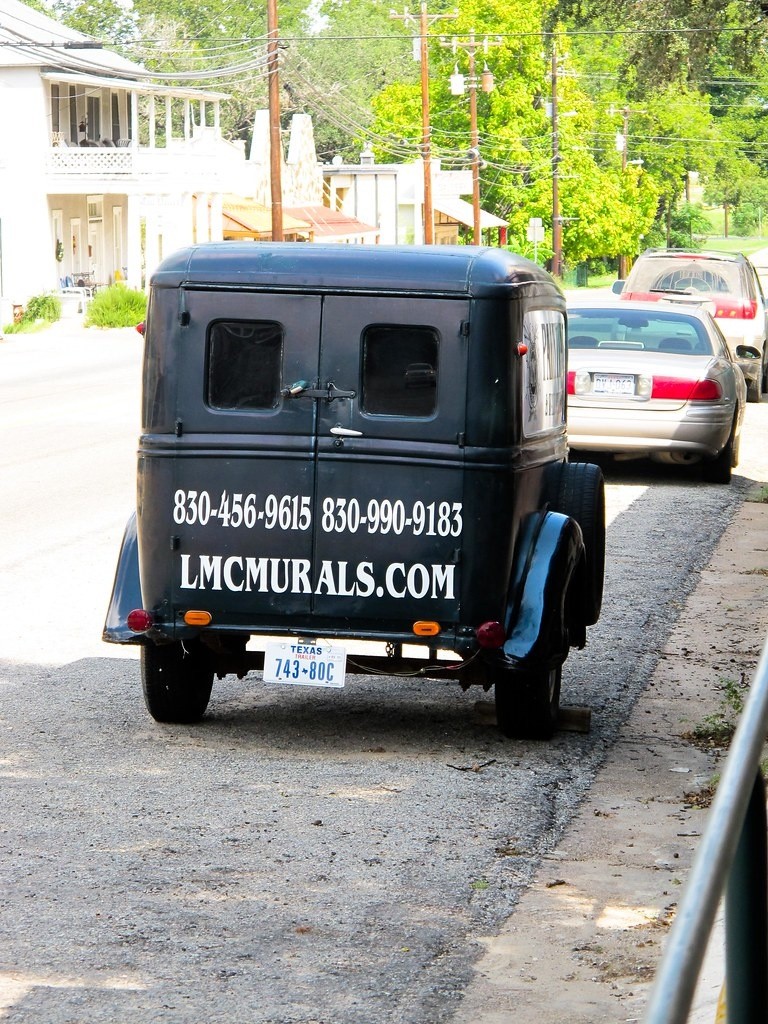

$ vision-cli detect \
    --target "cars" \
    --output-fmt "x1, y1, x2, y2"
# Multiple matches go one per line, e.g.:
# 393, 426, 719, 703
613, 248, 768, 402
562, 291, 748, 483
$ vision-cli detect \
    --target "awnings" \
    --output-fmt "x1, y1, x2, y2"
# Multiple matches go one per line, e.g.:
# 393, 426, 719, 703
193, 194, 309, 237
430, 199, 509, 230
282, 207, 381, 243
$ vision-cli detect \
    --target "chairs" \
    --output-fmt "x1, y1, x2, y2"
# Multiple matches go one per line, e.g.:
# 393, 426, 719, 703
658, 338, 692, 351
80, 139, 132, 147
568, 337, 599, 348
71, 270, 98, 296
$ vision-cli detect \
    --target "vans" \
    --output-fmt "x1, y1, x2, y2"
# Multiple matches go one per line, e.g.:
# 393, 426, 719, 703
101, 239, 604, 732
